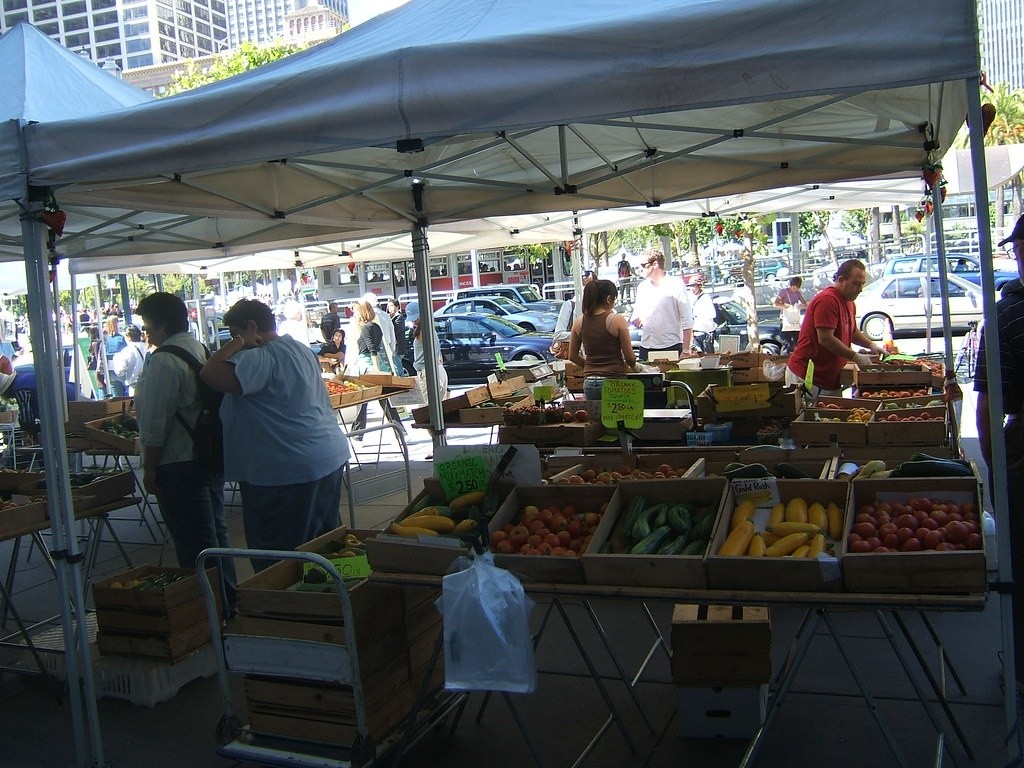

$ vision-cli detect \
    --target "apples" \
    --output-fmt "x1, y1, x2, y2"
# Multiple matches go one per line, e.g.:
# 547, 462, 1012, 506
492, 504, 602, 557
555, 463, 687, 484
847, 497, 983, 553
564, 410, 588, 422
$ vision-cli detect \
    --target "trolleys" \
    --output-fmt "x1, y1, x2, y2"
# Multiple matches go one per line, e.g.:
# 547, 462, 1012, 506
195, 548, 449, 768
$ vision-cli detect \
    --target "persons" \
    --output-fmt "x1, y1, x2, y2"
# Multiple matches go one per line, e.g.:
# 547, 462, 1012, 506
568, 280, 648, 400
61, 304, 159, 400
774, 276, 807, 355
630, 249, 693, 359
0, 353, 91, 431
201, 299, 351, 574
134, 292, 239, 619
972, 213, 1024, 684
278, 292, 409, 442
955, 259, 968, 271
918, 280, 939, 298
785, 259, 890, 396
405, 302, 447, 460
350, 263, 521, 287
686, 275, 716, 353
553, 271, 599, 360
617, 253, 639, 306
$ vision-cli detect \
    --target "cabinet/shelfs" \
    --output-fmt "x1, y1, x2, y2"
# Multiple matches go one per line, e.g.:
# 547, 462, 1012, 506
0, 422, 17, 471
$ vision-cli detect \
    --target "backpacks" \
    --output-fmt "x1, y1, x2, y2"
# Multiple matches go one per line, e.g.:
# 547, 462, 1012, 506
146, 344, 224, 472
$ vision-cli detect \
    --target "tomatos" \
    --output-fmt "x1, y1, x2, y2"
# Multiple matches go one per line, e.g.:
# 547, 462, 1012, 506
324, 381, 354, 396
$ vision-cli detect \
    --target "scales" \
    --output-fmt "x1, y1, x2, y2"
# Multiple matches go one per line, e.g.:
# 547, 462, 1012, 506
495, 359, 555, 381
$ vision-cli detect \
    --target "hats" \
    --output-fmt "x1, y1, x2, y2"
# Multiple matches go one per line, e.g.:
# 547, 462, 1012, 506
405, 302, 419, 322
998, 214, 1024, 247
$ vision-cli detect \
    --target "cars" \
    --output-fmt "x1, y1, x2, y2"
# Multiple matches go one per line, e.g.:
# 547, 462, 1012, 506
12, 312, 233, 375
853, 252, 1019, 341
673, 259, 851, 281
627, 296, 781, 354
378, 284, 566, 386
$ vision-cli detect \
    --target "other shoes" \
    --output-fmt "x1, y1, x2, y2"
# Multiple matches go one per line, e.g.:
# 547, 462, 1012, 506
425, 455, 432, 460
352, 436, 362, 441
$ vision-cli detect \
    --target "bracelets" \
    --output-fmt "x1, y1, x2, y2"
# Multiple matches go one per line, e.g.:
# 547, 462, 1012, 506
142, 463, 153, 467
681, 350, 691, 354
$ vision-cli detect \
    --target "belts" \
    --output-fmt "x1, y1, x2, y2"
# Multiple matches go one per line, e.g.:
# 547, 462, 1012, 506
587, 376, 622, 379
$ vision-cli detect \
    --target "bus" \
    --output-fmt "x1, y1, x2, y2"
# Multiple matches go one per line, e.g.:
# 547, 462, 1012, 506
316, 242, 576, 318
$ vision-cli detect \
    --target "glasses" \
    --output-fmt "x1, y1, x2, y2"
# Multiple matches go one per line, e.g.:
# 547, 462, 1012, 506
1006, 243, 1020, 260
640, 261, 654, 268
230, 326, 242, 338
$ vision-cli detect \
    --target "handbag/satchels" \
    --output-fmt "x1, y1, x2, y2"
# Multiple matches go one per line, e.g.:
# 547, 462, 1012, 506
310, 340, 337, 355
94, 372, 106, 389
552, 331, 571, 360
780, 302, 801, 332
87, 356, 97, 370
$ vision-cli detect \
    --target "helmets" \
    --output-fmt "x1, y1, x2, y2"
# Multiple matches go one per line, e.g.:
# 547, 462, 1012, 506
686, 276, 702, 287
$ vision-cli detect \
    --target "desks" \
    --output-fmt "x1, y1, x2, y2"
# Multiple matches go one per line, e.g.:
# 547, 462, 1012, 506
332, 388, 411, 530
364, 572, 988, 768
0, 498, 142, 707
84, 449, 242, 568
17, 443, 82, 473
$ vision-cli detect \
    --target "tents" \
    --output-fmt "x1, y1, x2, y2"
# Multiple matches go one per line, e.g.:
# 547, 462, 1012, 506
68, 142, 1024, 400
0, 0, 1021, 768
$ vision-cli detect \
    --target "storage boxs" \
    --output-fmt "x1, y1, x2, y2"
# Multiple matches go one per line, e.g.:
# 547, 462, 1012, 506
96, 612, 224, 659
85, 410, 140, 453
0, 410, 19, 423
0, 468, 138, 534
18, 611, 218, 707
90, 563, 219, 609
95, 589, 222, 635
69, 414, 119, 434
68, 397, 135, 415
222, 335, 987, 754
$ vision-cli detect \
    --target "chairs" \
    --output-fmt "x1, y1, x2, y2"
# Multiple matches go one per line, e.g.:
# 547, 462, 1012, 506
458, 327, 468, 338
468, 321, 478, 338
